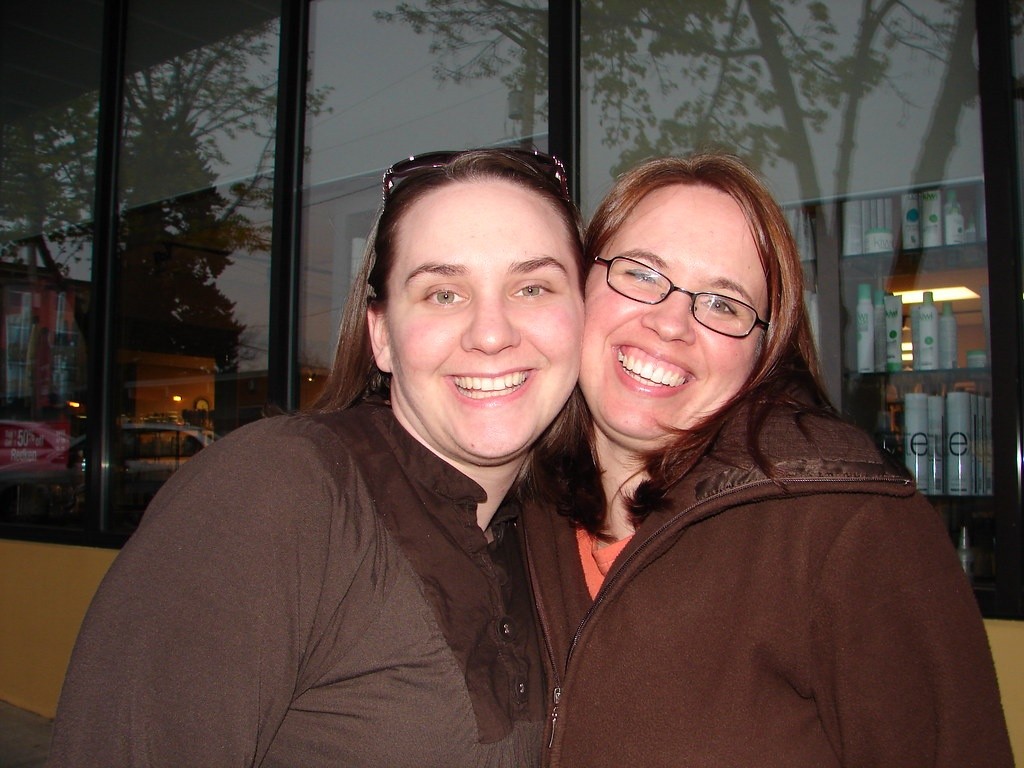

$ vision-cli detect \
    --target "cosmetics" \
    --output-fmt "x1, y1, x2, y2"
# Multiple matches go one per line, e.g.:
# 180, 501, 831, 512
779, 185, 990, 494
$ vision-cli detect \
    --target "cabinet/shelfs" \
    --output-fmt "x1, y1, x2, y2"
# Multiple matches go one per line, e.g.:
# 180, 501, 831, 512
777, 176, 998, 615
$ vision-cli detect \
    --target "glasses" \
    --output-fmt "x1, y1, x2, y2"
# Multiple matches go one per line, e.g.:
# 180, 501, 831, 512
595, 255, 769, 338
382, 147, 570, 222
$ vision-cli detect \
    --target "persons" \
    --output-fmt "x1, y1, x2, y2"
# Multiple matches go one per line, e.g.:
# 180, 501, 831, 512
40, 142, 589, 768
519, 155, 1016, 768
